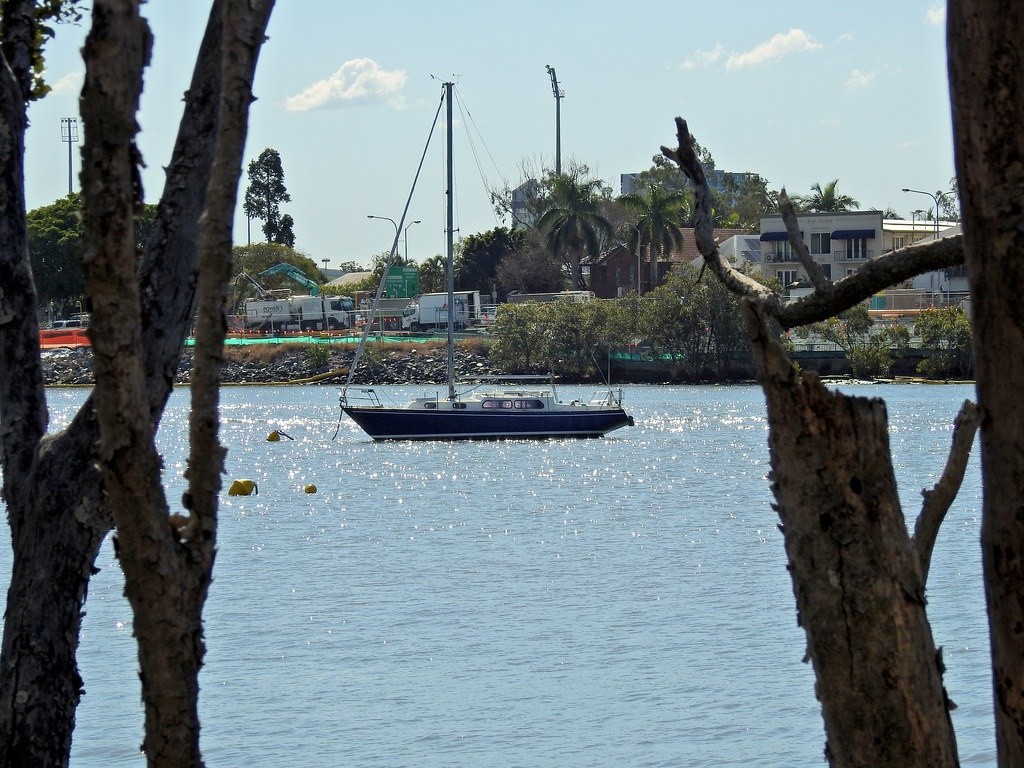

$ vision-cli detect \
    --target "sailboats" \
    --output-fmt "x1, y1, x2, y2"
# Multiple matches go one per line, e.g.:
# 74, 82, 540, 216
338, 74, 637, 442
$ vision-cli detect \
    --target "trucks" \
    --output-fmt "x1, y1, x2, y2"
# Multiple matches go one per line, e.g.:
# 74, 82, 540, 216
402, 290, 481, 332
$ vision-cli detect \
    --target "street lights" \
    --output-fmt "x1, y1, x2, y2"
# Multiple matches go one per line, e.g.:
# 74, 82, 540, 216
366, 215, 398, 259
899, 188, 958, 286
322, 257, 333, 282
404, 219, 421, 264
75, 299, 83, 327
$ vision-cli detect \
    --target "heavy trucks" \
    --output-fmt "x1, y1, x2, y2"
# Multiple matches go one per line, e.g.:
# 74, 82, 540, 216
244, 294, 355, 331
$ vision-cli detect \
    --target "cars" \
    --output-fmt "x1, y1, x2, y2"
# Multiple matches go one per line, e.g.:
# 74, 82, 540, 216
46, 319, 82, 328
